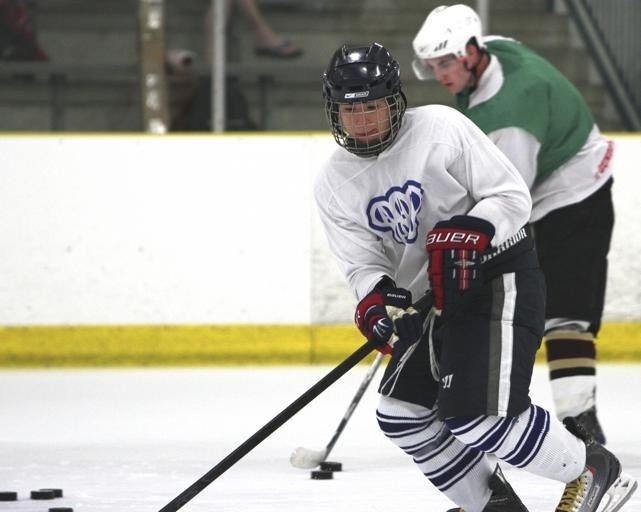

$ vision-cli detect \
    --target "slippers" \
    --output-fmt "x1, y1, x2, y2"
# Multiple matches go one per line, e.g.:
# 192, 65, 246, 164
249, 37, 306, 63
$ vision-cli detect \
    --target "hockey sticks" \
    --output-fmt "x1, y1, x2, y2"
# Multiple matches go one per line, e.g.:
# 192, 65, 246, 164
290, 352, 387, 469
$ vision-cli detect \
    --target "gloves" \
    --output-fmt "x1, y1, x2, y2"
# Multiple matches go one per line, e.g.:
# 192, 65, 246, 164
351, 281, 425, 355
423, 215, 495, 314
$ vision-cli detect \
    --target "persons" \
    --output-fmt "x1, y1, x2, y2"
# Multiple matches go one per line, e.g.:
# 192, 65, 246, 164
206, 0, 306, 60
411, 3, 618, 448
314, 40, 624, 511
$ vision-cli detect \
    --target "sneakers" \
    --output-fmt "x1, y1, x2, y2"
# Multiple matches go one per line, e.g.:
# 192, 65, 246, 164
553, 406, 623, 512
446, 462, 530, 512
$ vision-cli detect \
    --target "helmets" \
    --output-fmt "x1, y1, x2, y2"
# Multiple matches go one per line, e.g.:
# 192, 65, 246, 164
411, 3, 489, 88
319, 39, 408, 159
